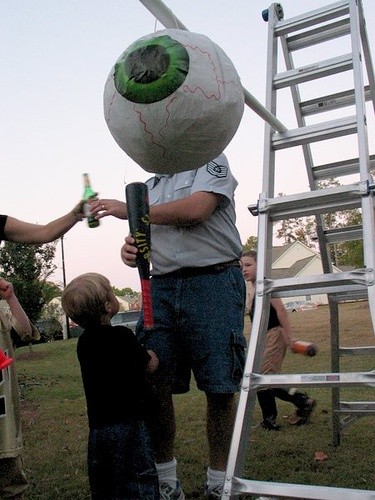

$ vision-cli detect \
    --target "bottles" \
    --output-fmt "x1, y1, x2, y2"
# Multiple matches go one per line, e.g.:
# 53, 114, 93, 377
82, 172, 101, 229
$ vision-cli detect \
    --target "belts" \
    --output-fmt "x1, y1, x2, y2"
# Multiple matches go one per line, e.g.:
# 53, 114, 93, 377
152, 259, 240, 278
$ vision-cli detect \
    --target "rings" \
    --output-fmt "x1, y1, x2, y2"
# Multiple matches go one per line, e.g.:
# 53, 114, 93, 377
101, 205, 105, 209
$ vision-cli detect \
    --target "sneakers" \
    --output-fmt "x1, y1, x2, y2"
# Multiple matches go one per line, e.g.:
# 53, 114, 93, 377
290, 396, 316, 426
157, 480, 184, 500
203, 483, 224, 500
250, 419, 278, 430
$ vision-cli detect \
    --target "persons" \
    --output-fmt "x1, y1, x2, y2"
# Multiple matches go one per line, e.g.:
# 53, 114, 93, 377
0, 276, 41, 500
0, 191, 99, 246
61, 272, 159, 500
90, 150, 246, 500
240, 251, 317, 432
